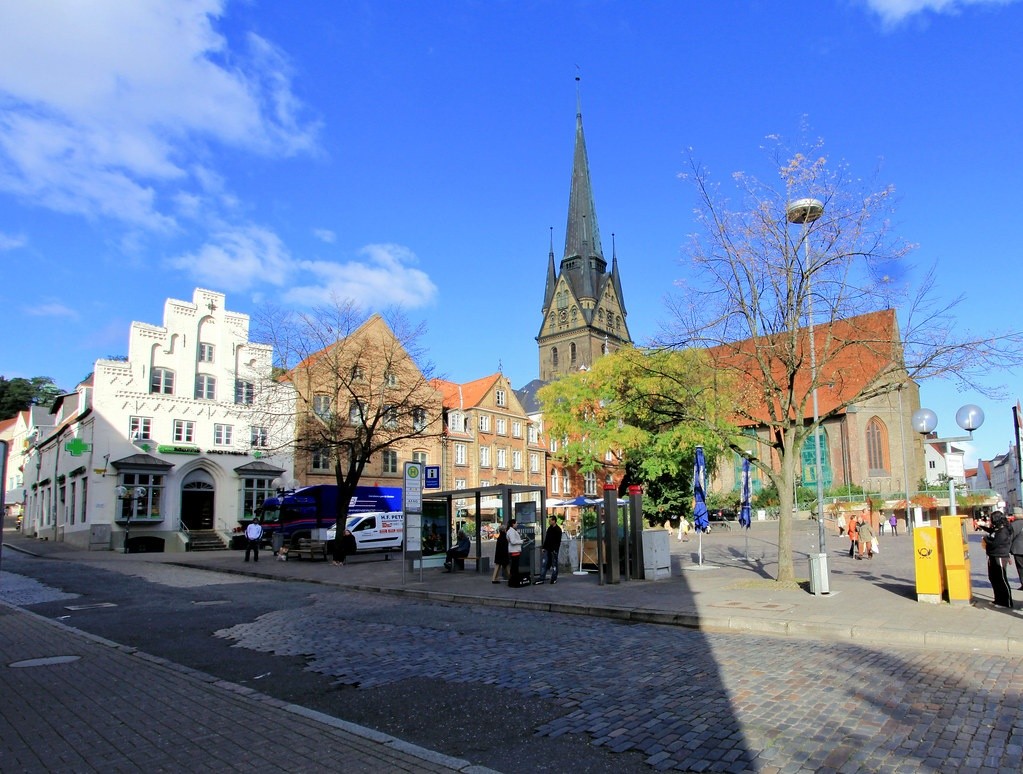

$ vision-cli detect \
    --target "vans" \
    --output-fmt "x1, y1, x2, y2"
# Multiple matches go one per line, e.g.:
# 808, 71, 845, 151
326, 512, 409, 552
707, 508, 739, 522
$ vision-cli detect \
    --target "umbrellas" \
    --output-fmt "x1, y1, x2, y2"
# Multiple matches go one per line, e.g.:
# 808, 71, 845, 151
731, 460, 762, 562
681, 445, 720, 571
535, 499, 577, 507
460, 499, 516, 523
554, 495, 597, 527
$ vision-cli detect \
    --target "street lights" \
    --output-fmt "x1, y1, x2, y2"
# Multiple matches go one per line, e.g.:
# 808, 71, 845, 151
783, 198, 833, 596
911, 404, 985, 516
271, 477, 300, 558
113, 485, 146, 553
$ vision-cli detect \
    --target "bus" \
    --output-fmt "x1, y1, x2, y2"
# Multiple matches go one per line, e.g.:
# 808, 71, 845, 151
249, 483, 404, 551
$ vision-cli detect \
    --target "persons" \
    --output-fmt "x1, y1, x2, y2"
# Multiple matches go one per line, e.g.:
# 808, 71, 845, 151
244, 518, 263, 562
847, 515, 860, 559
444, 529, 470, 571
878, 512, 886, 536
837, 513, 849, 537
855, 516, 876, 560
889, 514, 898, 536
492, 524, 511, 584
1008, 507, 1023, 591
532, 516, 562, 585
506, 519, 525, 578
329, 530, 357, 566
976, 511, 1013, 609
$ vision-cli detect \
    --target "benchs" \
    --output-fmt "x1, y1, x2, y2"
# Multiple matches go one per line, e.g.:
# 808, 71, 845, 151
451, 556, 492, 574
286, 538, 327, 562
340, 547, 402, 566
